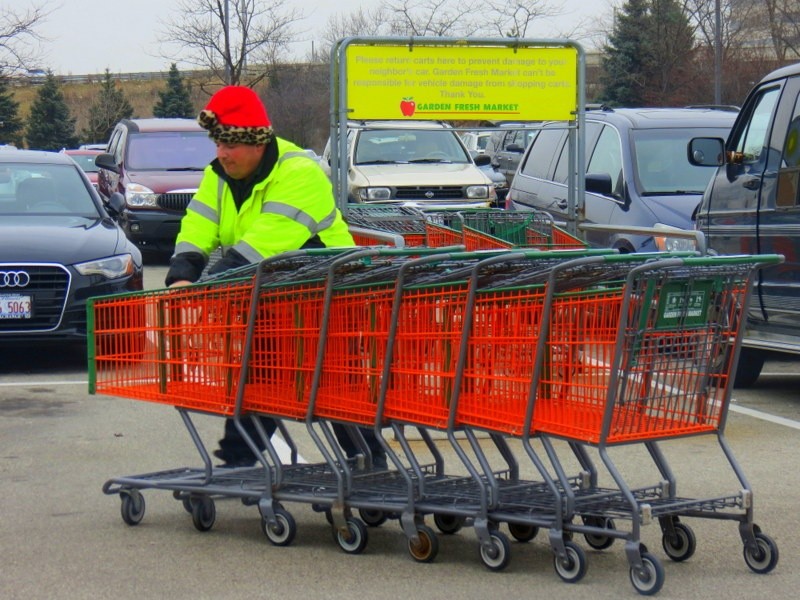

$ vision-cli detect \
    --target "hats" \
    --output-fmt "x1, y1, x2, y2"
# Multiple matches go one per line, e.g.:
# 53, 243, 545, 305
197, 86, 274, 144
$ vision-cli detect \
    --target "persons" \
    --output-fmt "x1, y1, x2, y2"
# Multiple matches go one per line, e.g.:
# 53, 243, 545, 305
166, 85, 390, 471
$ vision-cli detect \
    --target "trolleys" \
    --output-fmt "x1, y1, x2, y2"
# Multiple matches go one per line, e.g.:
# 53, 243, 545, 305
87, 203, 781, 597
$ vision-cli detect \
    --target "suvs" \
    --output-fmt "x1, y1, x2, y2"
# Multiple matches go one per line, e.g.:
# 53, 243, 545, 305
95, 118, 219, 245
506, 103, 744, 353
463, 118, 542, 188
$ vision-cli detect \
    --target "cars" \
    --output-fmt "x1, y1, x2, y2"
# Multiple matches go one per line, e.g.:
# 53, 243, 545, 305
0, 148, 148, 367
59, 144, 108, 195
318, 119, 509, 221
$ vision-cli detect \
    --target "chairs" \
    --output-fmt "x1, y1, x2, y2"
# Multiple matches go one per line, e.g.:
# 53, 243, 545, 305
16, 178, 55, 211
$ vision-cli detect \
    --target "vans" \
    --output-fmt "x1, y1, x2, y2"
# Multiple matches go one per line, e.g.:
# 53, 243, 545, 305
686, 62, 800, 385
